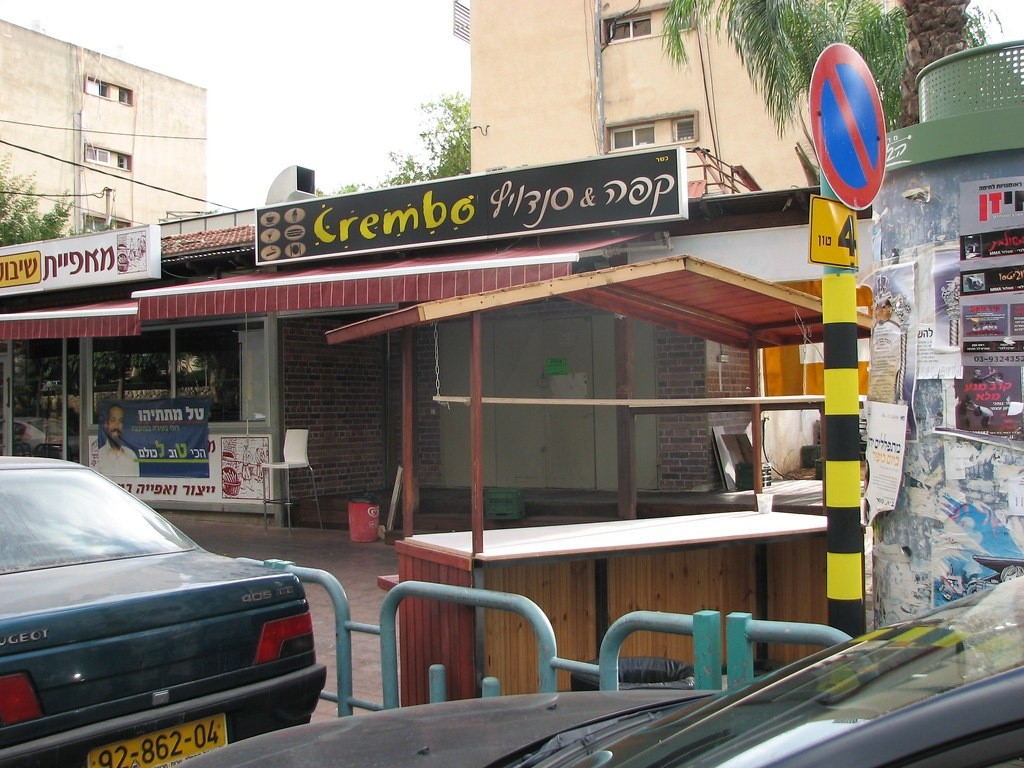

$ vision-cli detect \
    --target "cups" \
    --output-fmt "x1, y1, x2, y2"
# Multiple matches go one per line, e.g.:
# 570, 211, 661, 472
756, 494, 773, 514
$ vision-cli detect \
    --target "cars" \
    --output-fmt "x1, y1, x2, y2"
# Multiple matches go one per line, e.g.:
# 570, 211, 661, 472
0, 454, 330, 767
151, 570, 1023, 768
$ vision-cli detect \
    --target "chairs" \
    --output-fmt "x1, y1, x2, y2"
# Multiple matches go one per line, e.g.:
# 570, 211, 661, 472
261, 428, 323, 539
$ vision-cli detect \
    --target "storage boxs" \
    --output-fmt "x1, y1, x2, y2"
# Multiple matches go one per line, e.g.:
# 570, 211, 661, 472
483, 486, 527, 520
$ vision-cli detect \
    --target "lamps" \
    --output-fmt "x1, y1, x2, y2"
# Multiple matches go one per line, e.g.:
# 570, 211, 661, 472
898, 188, 933, 204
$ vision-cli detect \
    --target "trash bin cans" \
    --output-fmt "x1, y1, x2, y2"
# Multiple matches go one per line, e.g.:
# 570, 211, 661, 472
722, 655, 790, 677
571, 656, 695, 691
348, 492, 382, 542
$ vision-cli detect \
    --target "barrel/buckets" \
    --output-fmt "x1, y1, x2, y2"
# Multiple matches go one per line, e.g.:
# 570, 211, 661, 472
348, 501, 380, 543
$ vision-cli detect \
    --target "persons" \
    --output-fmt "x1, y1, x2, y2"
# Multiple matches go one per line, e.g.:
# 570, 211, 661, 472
95, 405, 140, 476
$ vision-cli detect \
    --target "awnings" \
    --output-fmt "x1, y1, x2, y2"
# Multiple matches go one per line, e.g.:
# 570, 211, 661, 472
0, 300, 142, 340
131, 231, 644, 321
